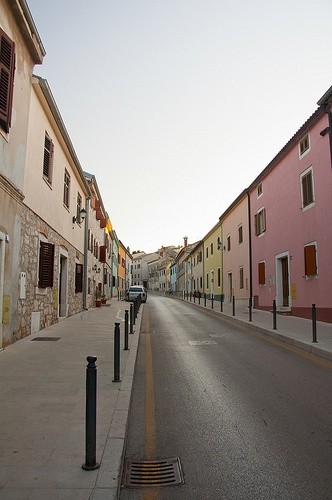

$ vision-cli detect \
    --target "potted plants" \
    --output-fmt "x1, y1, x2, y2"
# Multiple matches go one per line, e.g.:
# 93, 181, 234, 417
101, 294, 107, 304
95, 291, 102, 307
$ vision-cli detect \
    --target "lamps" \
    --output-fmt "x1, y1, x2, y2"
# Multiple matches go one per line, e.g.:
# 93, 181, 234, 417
73, 208, 87, 223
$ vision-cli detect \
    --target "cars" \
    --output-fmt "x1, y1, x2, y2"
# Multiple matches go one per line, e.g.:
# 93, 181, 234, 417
125, 286, 147, 303
